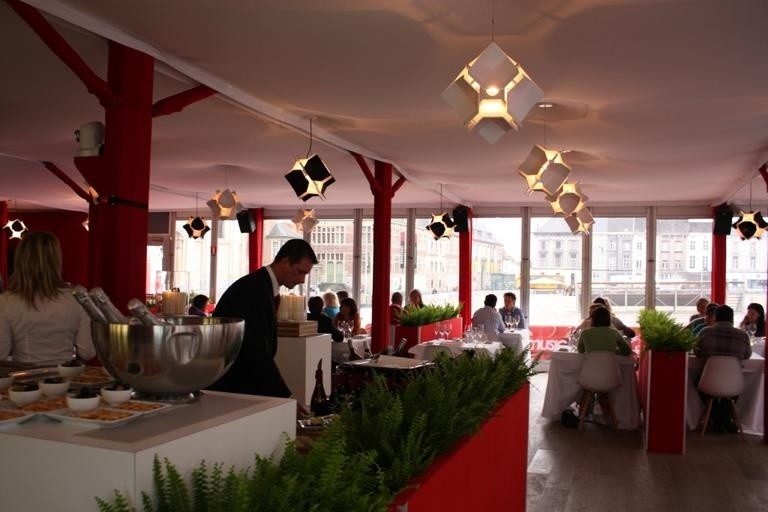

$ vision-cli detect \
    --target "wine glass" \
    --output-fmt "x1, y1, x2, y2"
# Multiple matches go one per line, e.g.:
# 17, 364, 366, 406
504, 315, 520, 332
749, 323, 757, 340
338, 321, 346, 334
568, 327, 583, 346
348, 320, 354, 338
433, 321, 484, 347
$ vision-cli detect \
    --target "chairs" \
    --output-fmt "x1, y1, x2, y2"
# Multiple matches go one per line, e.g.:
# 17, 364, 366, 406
696, 355, 748, 442
576, 350, 624, 436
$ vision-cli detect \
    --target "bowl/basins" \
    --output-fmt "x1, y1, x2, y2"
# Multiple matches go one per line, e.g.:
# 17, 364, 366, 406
8, 363, 133, 412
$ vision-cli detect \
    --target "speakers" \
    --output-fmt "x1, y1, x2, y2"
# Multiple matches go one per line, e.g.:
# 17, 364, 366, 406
713, 205, 733, 235
452, 207, 469, 232
237, 211, 256, 232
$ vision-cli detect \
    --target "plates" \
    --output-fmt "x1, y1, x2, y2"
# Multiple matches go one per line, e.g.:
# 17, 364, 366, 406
0, 365, 173, 429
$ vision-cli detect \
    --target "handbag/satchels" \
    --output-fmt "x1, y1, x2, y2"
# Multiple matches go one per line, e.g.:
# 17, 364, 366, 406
562, 409, 580, 427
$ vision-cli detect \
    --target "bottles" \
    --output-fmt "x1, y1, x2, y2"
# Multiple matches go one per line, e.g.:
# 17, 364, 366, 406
72, 284, 169, 324
311, 370, 328, 418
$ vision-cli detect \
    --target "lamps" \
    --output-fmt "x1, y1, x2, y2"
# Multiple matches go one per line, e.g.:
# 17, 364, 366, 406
517, 102, 573, 197
731, 181, 768, 242
183, 192, 210, 239
2, 198, 29, 241
207, 166, 242, 220
291, 202, 319, 235
566, 202, 597, 236
425, 186, 460, 241
441, 0, 545, 145
284, 115, 336, 203
544, 147, 589, 218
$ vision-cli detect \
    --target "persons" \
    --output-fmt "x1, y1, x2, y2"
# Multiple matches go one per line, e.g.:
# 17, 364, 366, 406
577, 307, 631, 426
305, 290, 362, 343
188, 295, 208, 319
573, 303, 614, 337
389, 292, 409, 328
584, 298, 635, 338
404, 289, 427, 316
681, 298, 768, 434
498, 293, 525, 330
0, 234, 96, 371
211, 239, 318, 418
472, 294, 505, 340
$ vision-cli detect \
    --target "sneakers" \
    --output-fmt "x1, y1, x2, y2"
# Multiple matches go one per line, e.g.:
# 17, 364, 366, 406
583, 414, 594, 421
